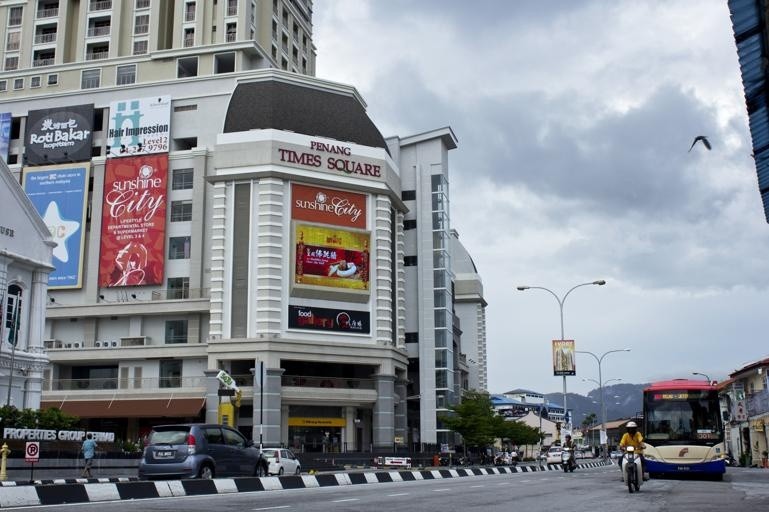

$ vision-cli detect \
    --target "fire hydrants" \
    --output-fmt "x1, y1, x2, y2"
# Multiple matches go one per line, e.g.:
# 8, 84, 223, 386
0, 442, 11, 481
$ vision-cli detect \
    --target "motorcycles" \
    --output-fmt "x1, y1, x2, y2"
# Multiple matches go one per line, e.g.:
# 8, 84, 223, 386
560, 447, 576, 472
616, 446, 650, 493
512, 458, 519, 467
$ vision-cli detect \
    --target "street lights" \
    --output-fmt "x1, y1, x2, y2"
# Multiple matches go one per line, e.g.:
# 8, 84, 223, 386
516, 280, 631, 461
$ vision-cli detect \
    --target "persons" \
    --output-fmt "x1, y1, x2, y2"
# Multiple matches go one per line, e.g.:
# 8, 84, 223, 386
510, 449, 518, 467
80, 433, 103, 478
562, 434, 577, 469
618, 421, 648, 482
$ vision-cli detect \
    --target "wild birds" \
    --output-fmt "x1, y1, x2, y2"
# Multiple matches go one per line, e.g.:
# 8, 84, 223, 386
689, 134, 712, 153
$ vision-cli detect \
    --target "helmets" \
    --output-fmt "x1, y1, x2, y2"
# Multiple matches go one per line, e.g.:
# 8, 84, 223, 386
626, 422, 637, 428
565, 435, 571, 438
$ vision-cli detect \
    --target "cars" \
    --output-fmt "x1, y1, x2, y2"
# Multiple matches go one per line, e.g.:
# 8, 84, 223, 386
547, 446, 563, 464
494, 451, 512, 466
138, 422, 302, 481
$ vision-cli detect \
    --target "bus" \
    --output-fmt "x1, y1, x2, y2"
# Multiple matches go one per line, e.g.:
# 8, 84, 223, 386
635, 379, 732, 481
